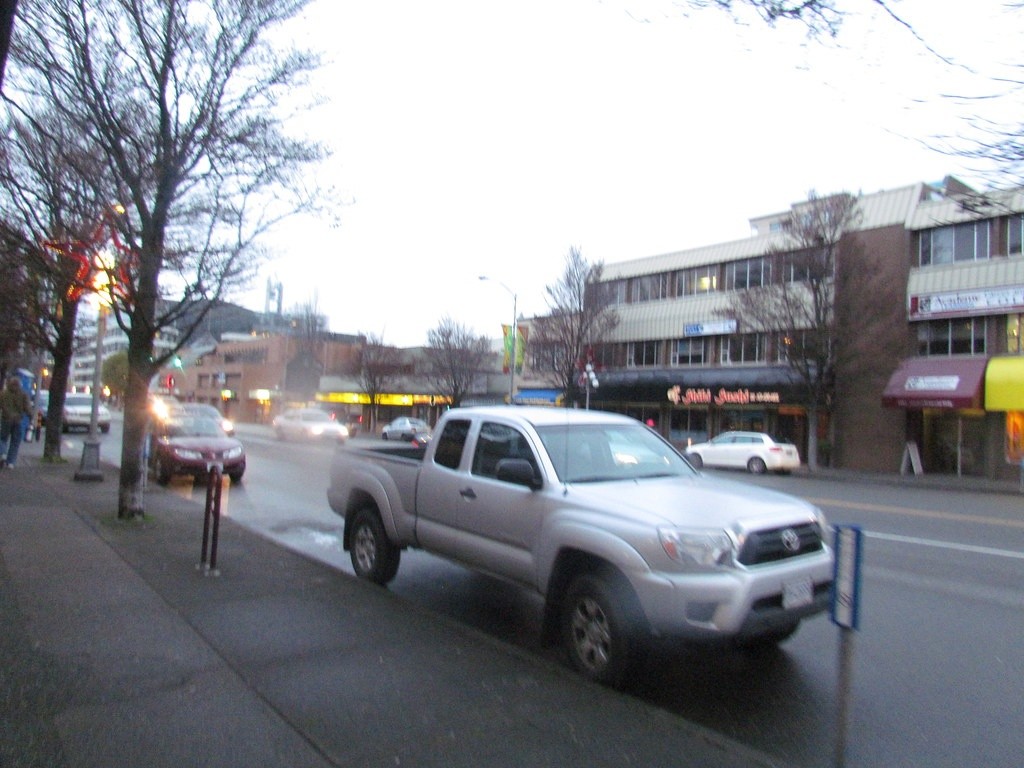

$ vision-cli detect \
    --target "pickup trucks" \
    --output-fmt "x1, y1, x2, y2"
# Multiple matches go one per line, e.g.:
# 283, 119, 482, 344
323, 402, 836, 688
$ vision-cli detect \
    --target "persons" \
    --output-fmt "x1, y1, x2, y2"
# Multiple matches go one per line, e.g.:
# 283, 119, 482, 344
0, 377, 35, 469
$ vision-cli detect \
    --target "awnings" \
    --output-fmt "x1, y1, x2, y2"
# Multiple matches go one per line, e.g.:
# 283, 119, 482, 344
983, 356, 1023, 412
577, 367, 832, 407
881, 356, 990, 408
504, 390, 568, 406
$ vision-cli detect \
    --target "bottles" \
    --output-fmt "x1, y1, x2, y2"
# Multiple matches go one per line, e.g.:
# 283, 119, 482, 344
25, 424, 34, 443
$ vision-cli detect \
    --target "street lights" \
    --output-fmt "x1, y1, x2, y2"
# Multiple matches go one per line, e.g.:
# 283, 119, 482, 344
478, 274, 518, 404
73, 249, 116, 482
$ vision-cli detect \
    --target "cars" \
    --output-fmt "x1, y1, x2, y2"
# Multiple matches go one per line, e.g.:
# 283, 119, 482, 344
682, 430, 801, 476
380, 414, 433, 442
38, 390, 111, 434
272, 406, 350, 447
144, 394, 247, 488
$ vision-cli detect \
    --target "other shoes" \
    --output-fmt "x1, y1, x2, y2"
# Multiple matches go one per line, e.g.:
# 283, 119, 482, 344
7, 463, 14, 469
0, 455, 6, 467
